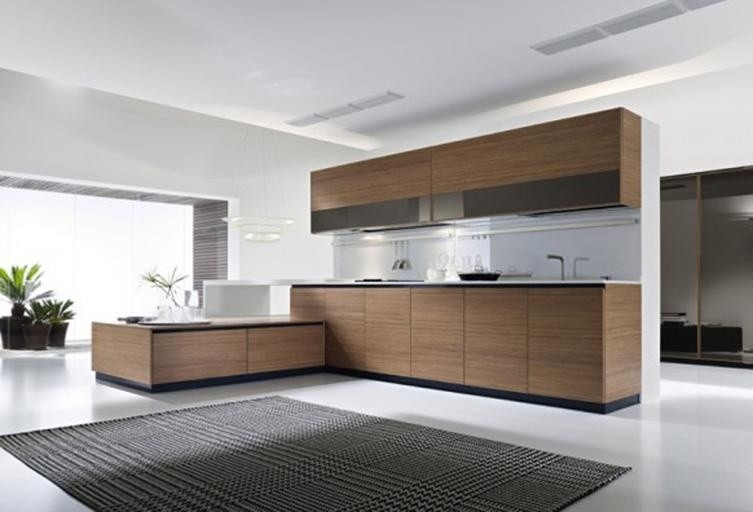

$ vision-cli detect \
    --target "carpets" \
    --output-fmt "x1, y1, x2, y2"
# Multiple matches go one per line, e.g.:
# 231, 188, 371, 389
0, 395, 633, 512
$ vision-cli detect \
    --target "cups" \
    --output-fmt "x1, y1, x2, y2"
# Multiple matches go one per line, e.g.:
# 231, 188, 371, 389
155, 305, 206, 324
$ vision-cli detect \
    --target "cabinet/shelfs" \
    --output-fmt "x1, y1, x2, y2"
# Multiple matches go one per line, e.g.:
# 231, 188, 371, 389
311, 146, 431, 235
432, 106, 642, 223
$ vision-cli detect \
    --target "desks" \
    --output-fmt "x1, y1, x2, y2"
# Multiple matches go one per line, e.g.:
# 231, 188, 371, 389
91, 315, 326, 393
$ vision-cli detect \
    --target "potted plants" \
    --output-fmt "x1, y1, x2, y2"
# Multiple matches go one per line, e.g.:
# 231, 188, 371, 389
0, 265, 76, 350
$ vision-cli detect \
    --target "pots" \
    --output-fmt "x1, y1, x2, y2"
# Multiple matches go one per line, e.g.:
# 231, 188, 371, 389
455, 269, 533, 281
